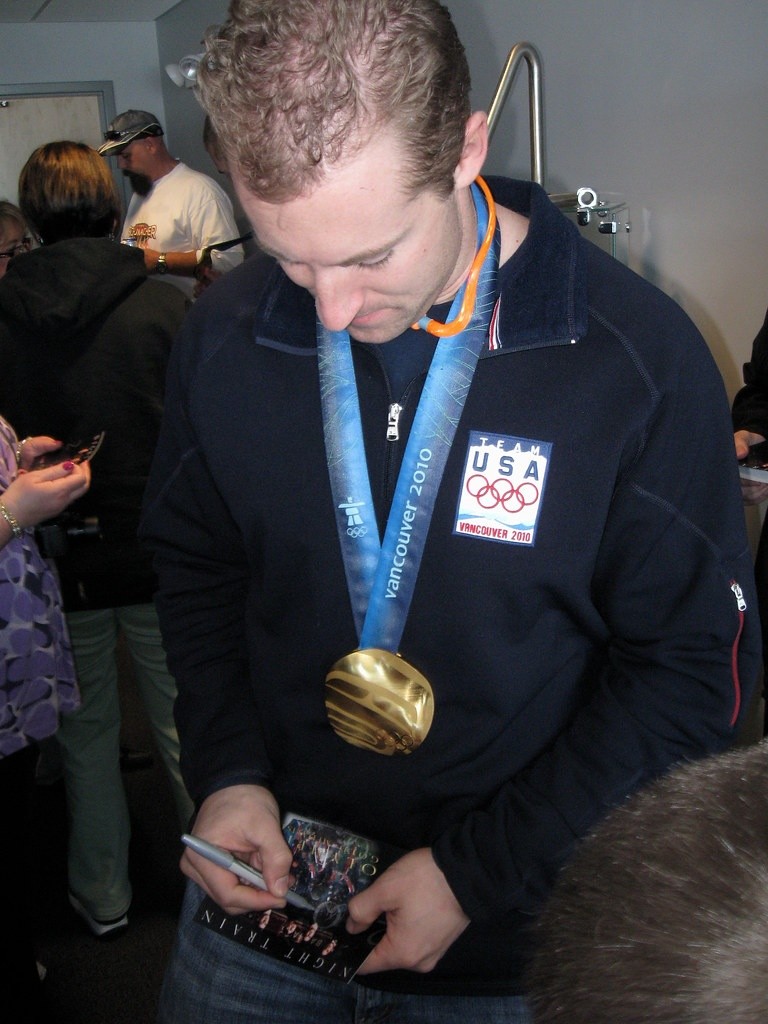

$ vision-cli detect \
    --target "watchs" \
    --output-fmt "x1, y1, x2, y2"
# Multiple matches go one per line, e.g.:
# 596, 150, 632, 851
156, 252, 167, 273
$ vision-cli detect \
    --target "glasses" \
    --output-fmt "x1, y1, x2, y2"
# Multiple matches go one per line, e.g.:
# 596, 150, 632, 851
103, 130, 154, 142
0, 237, 33, 257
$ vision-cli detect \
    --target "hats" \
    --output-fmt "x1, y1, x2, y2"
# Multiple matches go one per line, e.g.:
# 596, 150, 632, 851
97, 109, 164, 157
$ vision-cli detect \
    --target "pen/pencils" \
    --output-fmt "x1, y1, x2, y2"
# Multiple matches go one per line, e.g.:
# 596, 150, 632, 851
180, 833, 318, 912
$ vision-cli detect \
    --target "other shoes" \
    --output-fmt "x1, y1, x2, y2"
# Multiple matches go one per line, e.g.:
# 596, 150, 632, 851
68, 890, 129, 938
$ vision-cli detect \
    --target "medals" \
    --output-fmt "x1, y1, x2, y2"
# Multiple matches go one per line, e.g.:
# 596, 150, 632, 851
325, 648, 434, 755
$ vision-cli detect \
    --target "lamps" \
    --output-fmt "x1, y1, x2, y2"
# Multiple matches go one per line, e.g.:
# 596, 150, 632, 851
165, 52, 213, 88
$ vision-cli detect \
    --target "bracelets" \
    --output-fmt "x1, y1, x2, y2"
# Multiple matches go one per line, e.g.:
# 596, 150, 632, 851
1, 502, 22, 538
16, 437, 33, 464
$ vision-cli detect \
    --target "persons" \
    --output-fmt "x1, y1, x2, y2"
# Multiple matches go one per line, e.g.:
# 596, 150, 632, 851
730, 305, 768, 735
1, 109, 258, 935
515, 733, 768, 1024
142, 0, 760, 1024
0, 419, 105, 1024
